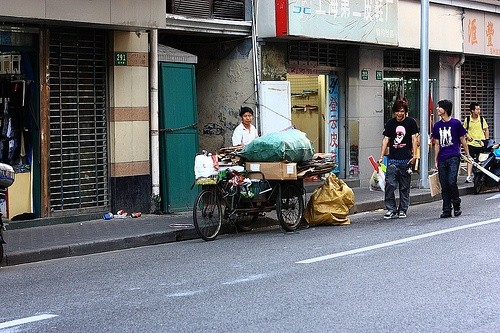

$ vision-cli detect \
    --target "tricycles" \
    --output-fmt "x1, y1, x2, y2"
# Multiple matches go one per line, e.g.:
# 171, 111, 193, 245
193, 153, 305, 241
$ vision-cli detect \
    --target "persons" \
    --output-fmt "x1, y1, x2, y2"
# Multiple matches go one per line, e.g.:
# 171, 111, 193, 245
464, 103, 489, 182
378, 101, 418, 218
231, 107, 258, 146
432, 99, 474, 218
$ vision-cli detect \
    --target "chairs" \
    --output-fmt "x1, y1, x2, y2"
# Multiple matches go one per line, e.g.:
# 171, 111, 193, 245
457, 144, 468, 182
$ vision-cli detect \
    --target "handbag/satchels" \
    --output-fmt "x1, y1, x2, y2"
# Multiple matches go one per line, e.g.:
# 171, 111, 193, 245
428, 169, 442, 197
369, 165, 385, 192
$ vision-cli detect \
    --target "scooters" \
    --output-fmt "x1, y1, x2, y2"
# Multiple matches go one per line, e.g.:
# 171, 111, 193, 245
472, 139, 500, 195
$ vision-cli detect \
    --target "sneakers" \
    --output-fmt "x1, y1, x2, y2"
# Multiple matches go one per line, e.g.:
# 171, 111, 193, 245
465, 176, 472, 183
454, 209, 462, 217
440, 212, 452, 218
398, 210, 406, 219
384, 210, 397, 219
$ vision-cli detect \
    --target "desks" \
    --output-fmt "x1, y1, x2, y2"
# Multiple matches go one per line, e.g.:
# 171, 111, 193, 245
0, 172, 31, 221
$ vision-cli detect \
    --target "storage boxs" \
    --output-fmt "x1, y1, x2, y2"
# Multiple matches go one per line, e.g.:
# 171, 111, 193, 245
246, 160, 297, 180
